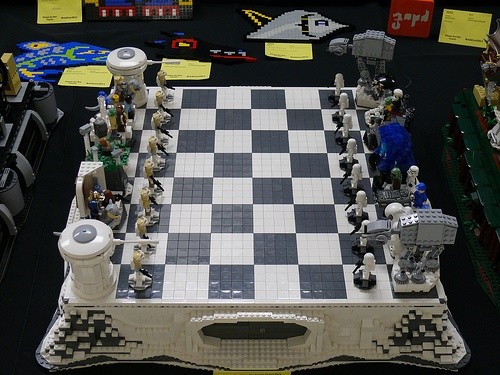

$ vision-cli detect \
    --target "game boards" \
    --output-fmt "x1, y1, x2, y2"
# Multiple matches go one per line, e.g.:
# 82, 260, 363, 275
36, 85, 472, 372
